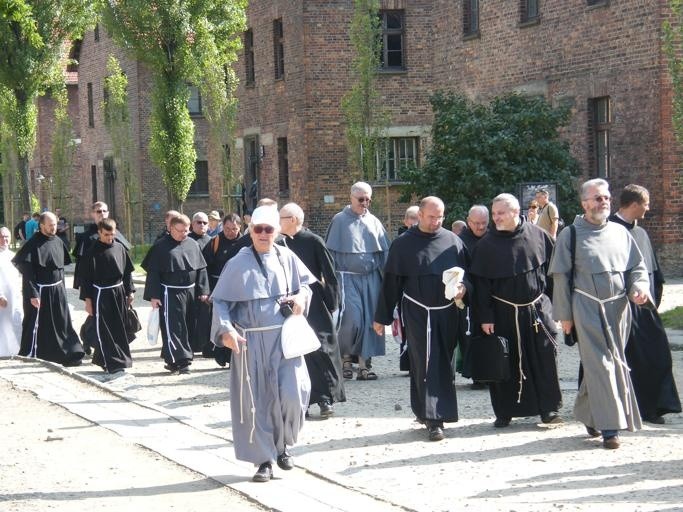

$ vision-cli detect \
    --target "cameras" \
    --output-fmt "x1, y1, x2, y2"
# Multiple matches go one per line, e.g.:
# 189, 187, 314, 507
280, 303, 293, 318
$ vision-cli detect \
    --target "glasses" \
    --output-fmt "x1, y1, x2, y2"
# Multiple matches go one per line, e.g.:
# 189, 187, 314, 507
94, 209, 294, 237
427, 195, 613, 227
351, 193, 370, 203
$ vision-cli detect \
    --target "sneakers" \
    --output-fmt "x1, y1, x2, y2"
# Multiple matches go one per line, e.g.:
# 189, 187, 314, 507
494, 413, 665, 450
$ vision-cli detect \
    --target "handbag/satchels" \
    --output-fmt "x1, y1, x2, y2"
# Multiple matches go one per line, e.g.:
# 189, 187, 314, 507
126, 303, 143, 333
564, 325, 576, 346
462, 329, 510, 384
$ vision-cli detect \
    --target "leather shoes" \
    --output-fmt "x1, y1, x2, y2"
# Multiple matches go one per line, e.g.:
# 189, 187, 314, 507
252, 359, 445, 481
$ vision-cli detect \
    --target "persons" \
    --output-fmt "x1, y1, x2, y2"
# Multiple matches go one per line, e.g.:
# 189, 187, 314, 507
606, 182, 683, 425
206, 207, 318, 484
325, 181, 562, 416
459, 192, 574, 427
1, 201, 143, 374
372, 196, 473, 440
140, 195, 347, 417
547, 177, 650, 447
